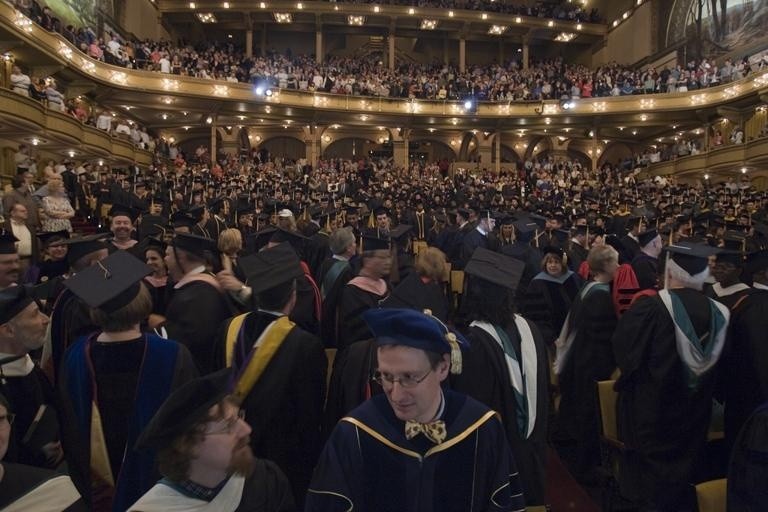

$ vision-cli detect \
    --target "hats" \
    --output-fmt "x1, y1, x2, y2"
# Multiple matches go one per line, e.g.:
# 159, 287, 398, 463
167, 231, 216, 256
457, 207, 472, 217
661, 239, 727, 277
169, 208, 203, 226
308, 203, 323, 216
0, 283, 37, 325
187, 204, 206, 224
429, 180, 456, 211
35, 229, 70, 240
458, 183, 497, 207
359, 308, 472, 375
498, 183, 544, 210
147, 197, 165, 212
464, 245, 526, 291
208, 198, 224, 206
356, 234, 400, 251
626, 192, 655, 216
137, 234, 168, 258
246, 224, 314, 246
373, 205, 389, 216
588, 186, 626, 225
330, 183, 367, 206
0, 235, 21, 254
155, 159, 237, 198
545, 202, 588, 226
133, 364, 233, 458
715, 249, 744, 265
237, 171, 305, 202
704, 193, 768, 234
107, 203, 138, 219
512, 216, 540, 234
316, 211, 338, 232
479, 210, 503, 219
391, 179, 429, 223
637, 228, 658, 248
305, 188, 329, 203
341, 207, 364, 210
575, 224, 601, 234
238, 240, 305, 309
446, 212, 457, 217
740, 248, 768, 273
551, 227, 574, 235
367, 180, 390, 205
543, 245, 568, 267
271, 204, 301, 212
625, 216, 647, 233
655, 194, 704, 238
233, 207, 252, 214
62, 248, 156, 313
435, 214, 446, 223
492, 215, 519, 225
386, 224, 415, 242
723, 234, 744, 250
61, 156, 153, 202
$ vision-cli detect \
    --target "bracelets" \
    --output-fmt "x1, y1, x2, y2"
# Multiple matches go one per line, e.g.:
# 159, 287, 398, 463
235, 283, 249, 295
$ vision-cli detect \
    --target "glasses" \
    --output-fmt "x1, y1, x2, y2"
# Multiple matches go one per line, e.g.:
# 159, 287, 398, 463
712, 265, 737, 270
373, 360, 438, 388
48, 244, 66, 247
0, 413, 16, 430
374, 255, 393, 260
190, 408, 246, 435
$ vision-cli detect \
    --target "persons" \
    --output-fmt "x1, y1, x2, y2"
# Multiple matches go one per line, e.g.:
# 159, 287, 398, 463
123, 40, 135, 60
324, 247, 456, 437
47, 238, 109, 380
116, 119, 130, 134
64, 156, 154, 237
43, 160, 60, 182
139, 126, 150, 146
0, 287, 52, 406
91, 38, 101, 59
97, 110, 113, 133
145, 238, 168, 312
14, 145, 32, 173
168, 236, 233, 327
4, 174, 38, 254
214, 247, 312, 410
537, 125, 766, 243
123, 367, 294, 512
131, 123, 140, 142
41, 7, 56, 30
78, 25, 96, 50
0, 230, 25, 288
395, 248, 456, 324
64, 249, 199, 465
218, 243, 312, 298
0, 391, 88, 512
42, 174, 75, 236
303, 309, 524, 511
10, 65, 31, 97
164, 241, 185, 287
65, 25, 75, 43
39, 77, 49, 92
527, 251, 584, 322
334, 238, 394, 326
106, 31, 125, 63
320, 227, 356, 296
557, 245, 621, 397
458, 246, 548, 507
611, 242, 725, 446
31, 76, 40, 95
